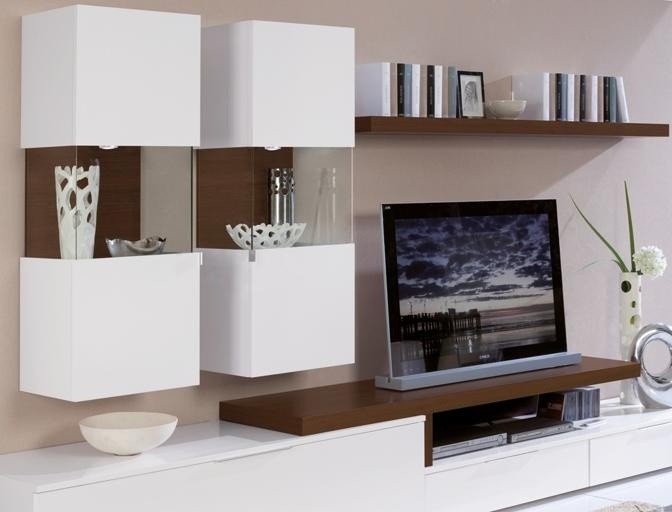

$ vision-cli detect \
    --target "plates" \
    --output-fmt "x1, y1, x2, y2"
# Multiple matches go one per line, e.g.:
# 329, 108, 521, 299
106, 235, 166, 255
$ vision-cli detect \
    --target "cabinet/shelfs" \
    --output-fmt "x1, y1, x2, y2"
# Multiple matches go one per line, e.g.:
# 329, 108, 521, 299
19, 4, 199, 403
200, 22, 356, 379
0, 358, 672, 512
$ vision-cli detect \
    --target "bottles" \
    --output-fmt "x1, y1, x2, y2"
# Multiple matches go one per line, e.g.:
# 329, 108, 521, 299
310, 165, 337, 246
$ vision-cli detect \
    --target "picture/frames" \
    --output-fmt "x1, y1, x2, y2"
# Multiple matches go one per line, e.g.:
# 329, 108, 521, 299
458, 70, 486, 120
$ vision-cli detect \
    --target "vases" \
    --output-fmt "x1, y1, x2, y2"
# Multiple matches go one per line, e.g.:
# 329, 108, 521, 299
619, 273, 641, 406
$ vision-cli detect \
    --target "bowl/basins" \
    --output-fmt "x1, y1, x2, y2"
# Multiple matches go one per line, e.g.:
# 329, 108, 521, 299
487, 100, 528, 120
78, 410, 179, 457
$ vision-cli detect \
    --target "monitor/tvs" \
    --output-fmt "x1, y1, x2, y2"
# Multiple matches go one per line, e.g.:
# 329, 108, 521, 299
373, 199, 584, 391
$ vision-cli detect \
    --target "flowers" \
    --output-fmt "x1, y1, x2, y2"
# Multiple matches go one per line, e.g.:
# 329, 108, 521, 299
568, 181, 668, 279
